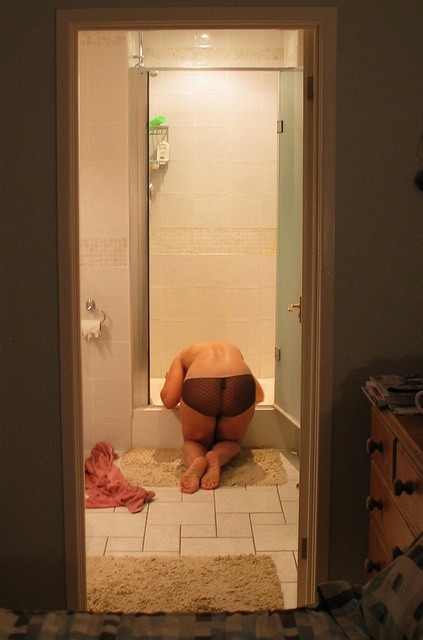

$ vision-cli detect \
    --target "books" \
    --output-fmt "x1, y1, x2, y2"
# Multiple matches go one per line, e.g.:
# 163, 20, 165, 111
360, 373, 423, 416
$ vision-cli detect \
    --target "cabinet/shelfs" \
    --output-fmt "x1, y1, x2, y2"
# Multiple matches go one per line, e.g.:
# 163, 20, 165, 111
364, 403, 423, 582
149, 125, 169, 170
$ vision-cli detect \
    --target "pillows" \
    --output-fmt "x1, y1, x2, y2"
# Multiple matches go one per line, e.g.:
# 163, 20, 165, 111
316, 530, 423, 640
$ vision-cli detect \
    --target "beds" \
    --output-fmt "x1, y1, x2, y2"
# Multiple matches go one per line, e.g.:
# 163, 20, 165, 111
1, 544, 350, 640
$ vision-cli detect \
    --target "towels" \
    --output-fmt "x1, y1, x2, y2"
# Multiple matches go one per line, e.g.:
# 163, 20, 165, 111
84, 441, 156, 513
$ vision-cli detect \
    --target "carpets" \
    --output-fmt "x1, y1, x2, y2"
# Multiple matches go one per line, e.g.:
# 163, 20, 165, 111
85, 554, 285, 614
120, 446, 288, 487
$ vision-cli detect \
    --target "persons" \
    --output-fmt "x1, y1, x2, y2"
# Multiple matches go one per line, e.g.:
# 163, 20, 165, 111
160, 341, 264, 494
